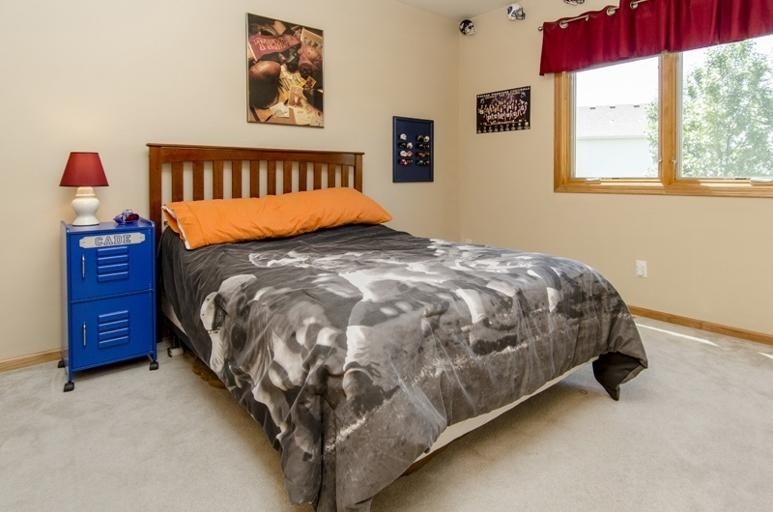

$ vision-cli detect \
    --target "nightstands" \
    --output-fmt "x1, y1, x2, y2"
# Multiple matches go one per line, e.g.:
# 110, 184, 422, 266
58, 215, 158, 384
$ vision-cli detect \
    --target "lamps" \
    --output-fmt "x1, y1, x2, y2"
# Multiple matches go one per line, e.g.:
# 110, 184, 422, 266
59, 151, 111, 227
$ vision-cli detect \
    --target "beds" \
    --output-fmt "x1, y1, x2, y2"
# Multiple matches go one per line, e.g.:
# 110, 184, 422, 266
147, 140, 615, 512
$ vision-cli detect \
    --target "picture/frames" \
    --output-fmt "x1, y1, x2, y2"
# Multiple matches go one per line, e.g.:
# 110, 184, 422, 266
392, 116, 434, 184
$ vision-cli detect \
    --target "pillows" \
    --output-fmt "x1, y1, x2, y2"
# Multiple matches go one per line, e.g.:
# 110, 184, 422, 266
161, 187, 393, 253
